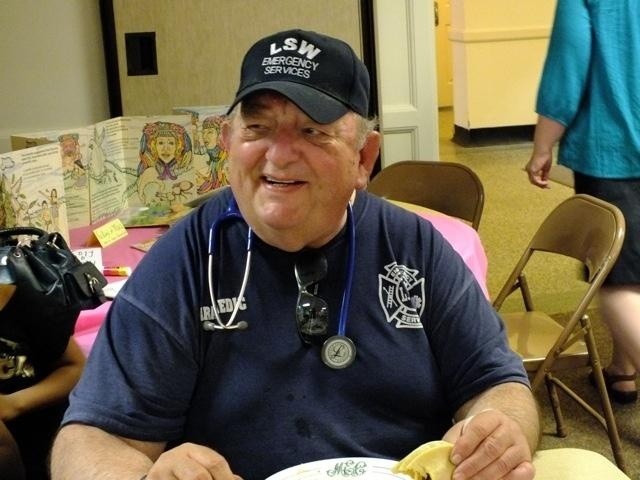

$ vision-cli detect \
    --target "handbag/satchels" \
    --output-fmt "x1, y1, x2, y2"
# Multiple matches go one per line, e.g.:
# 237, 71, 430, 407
1, 228, 108, 395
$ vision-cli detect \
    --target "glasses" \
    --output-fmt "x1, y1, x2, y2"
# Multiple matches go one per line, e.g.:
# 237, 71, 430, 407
294, 247, 328, 345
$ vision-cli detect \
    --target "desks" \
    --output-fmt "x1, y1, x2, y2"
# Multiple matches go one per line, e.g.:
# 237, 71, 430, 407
532, 448, 630, 480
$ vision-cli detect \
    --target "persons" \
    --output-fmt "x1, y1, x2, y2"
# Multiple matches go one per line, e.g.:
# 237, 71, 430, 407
0, 285, 88, 480
526, 1, 639, 403
47, 28, 541, 480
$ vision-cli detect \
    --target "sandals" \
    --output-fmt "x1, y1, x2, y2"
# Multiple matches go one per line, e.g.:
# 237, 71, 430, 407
587, 366, 638, 403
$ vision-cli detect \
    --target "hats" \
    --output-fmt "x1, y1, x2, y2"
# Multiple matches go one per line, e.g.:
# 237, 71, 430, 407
228, 30, 371, 125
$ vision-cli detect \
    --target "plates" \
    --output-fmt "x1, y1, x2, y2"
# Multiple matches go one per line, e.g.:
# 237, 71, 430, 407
265, 455, 413, 480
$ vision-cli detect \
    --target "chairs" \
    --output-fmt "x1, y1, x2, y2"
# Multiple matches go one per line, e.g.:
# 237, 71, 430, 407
493, 193, 627, 471
366, 161, 483, 229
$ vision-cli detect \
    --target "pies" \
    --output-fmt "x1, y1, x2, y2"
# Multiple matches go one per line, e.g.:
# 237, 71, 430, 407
391, 440, 457, 480
